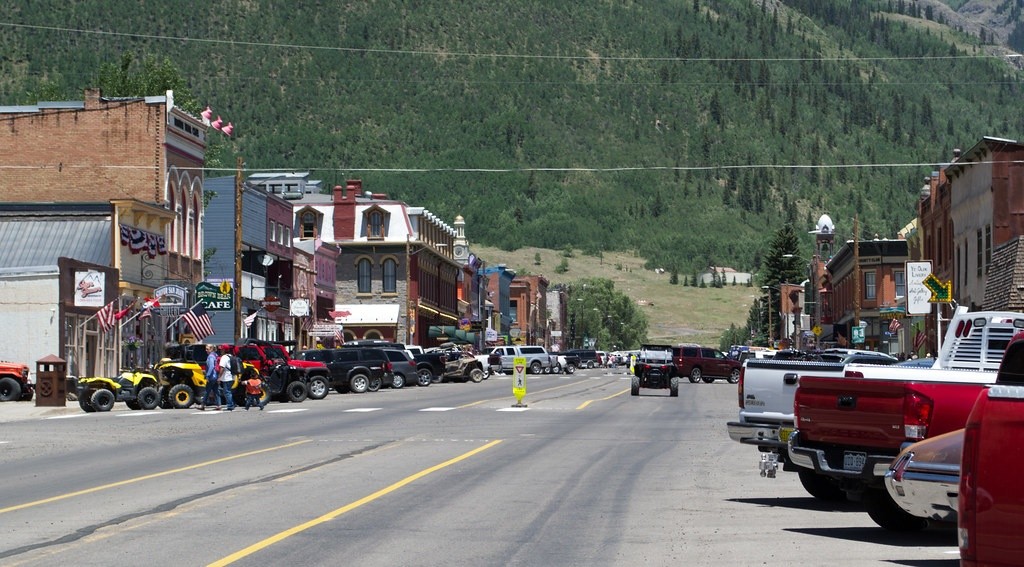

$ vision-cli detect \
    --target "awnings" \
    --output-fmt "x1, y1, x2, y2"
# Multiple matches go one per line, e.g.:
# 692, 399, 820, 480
428, 326, 476, 344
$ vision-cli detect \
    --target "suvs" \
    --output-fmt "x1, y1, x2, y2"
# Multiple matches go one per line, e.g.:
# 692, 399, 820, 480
631, 344, 679, 397
165, 341, 208, 370
671, 343, 742, 384
444, 348, 484, 383
216, 338, 332, 403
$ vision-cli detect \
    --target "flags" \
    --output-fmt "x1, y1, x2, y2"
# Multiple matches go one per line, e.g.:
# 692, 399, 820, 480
913, 328, 928, 351
304, 316, 314, 331
243, 307, 263, 328
889, 319, 904, 332
94, 298, 152, 333
183, 302, 216, 342
335, 329, 343, 344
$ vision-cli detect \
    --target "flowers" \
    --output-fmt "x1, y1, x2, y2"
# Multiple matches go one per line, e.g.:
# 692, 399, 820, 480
123, 336, 144, 348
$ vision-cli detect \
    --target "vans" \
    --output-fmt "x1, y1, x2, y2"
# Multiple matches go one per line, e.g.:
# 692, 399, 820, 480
297, 348, 386, 394
596, 351, 606, 365
569, 349, 602, 369
474, 345, 551, 375
727, 345, 780, 364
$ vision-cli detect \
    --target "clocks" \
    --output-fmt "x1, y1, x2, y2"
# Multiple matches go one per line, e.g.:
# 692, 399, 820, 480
453, 246, 465, 256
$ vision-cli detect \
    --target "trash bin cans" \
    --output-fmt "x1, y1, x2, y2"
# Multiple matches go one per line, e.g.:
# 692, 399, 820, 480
36, 354, 67, 407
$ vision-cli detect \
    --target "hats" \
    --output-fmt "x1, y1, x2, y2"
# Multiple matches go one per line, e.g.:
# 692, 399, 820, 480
218, 344, 229, 351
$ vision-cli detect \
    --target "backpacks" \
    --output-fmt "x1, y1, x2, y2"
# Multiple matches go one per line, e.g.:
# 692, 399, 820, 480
225, 354, 242, 374
209, 353, 222, 374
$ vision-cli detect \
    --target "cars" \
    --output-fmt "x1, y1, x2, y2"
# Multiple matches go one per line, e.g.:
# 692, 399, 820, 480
626, 352, 640, 368
382, 349, 418, 388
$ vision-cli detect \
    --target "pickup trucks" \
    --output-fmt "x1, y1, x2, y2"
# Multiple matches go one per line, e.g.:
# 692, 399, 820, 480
548, 352, 580, 374
473, 354, 502, 379
787, 375, 1023, 530
726, 306, 1024, 478
341, 339, 447, 387
958, 329, 1024, 567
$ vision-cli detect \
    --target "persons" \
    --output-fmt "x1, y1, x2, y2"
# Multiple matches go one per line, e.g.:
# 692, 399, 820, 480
598, 354, 622, 368
195, 344, 265, 412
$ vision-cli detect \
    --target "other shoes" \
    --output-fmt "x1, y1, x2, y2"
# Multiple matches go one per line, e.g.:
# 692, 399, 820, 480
214, 406, 223, 410
260, 406, 264, 410
242, 408, 247, 412
223, 408, 231, 411
194, 406, 204, 411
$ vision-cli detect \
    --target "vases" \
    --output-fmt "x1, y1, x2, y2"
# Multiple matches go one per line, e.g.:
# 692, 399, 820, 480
129, 345, 136, 350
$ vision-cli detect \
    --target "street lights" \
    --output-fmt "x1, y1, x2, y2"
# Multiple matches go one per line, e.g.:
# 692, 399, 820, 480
762, 286, 789, 344
783, 254, 817, 346
